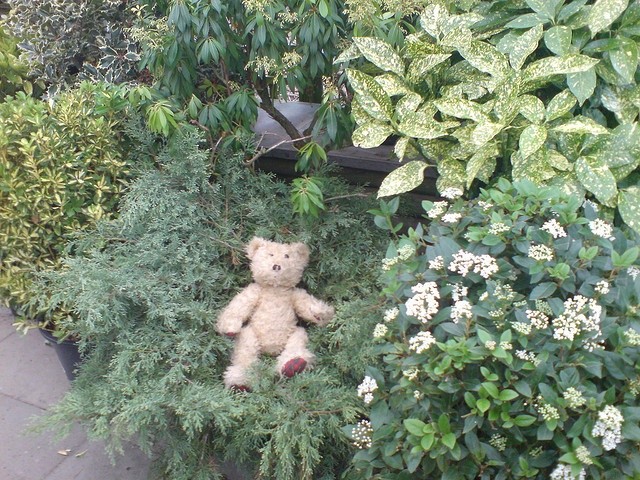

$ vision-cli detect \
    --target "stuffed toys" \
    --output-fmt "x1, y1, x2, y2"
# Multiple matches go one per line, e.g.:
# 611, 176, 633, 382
218, 235, 335, 391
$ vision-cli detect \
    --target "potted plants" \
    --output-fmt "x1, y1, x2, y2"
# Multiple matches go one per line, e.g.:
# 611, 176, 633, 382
0, 81, 135, 381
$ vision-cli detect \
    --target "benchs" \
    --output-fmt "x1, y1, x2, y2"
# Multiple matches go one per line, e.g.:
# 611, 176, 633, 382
252, 101, 462, 200
